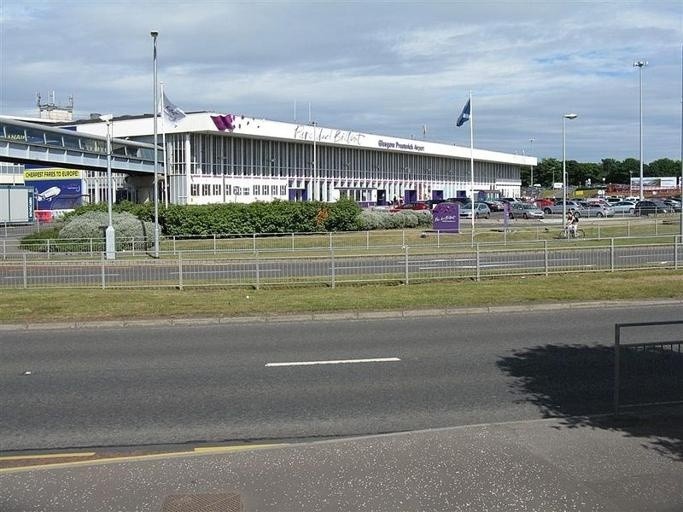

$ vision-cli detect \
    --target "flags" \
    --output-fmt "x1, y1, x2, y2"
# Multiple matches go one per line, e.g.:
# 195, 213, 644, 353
456, 99, 470, 127
157, 90, 187, 124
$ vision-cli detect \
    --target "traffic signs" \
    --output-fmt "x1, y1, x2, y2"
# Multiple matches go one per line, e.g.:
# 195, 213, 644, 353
561, 112, 579, 239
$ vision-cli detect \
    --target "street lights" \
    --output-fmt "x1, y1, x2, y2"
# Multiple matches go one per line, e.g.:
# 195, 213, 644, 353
149, 30, 160, 258
633, 58, 649, 202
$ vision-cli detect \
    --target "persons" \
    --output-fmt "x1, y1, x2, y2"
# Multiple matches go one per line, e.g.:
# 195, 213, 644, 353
567, 211, 579, 237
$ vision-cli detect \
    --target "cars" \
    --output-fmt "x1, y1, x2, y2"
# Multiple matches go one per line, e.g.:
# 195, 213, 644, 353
389, 193, 682, 220
